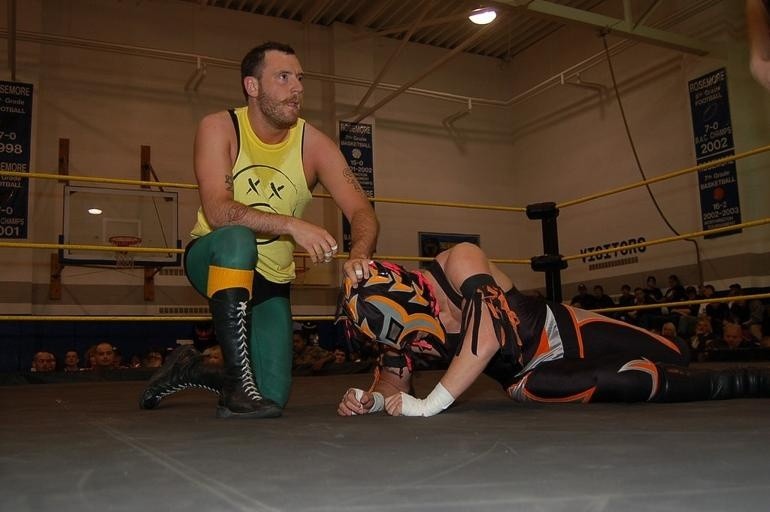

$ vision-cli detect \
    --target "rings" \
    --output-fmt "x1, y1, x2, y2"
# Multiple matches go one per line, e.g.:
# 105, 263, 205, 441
355, 270, 362, 276
324, 251, 332, 259
330, 243, 338, 252
324, 258, 332, 263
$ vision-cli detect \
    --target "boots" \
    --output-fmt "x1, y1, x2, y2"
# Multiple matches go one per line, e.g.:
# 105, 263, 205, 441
693, 363, 770, 402
140, 344, 225, 412
209, 287, 284, 419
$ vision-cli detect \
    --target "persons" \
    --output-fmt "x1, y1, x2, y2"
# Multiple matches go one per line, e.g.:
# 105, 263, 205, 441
331, 240, 770, 418
568, 274, 769, 374
137, 40, 382, 420
